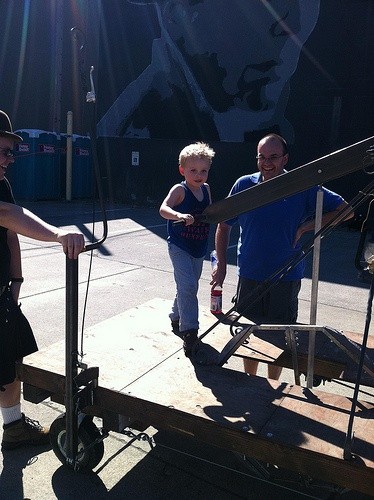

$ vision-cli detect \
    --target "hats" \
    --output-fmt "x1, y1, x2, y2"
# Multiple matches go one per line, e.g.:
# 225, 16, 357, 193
0, 110, 23, 142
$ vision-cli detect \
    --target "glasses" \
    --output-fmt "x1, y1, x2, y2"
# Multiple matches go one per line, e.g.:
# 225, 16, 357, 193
0, 148, 14, 157
256, 153, 285, 161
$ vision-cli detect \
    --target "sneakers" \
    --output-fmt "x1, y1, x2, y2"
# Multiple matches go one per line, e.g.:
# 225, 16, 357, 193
171, 319, 179, 333
183, 336, 198, 350
1, 413, 50, 450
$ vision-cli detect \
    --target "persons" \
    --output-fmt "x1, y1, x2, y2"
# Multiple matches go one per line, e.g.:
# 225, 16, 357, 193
159, 141, 216, 361
209, 133, 355, 382
0, 108, 85, 449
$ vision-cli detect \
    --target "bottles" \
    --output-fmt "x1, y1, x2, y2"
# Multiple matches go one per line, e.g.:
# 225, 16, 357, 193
211, 285, 222, 315
